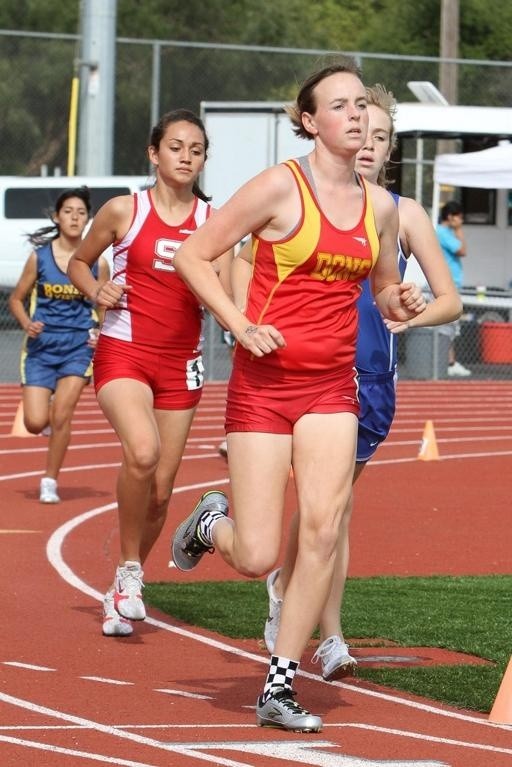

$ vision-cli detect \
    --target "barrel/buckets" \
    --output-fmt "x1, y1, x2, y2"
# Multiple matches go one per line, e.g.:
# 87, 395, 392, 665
481, 322, 512, 366
406, 325, 453, 382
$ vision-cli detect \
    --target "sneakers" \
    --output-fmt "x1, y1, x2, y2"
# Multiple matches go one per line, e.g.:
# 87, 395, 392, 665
263, 568, 284, 656
447, 361, 471, 377
255, 689, 323, 734
113, 561, 147, 621
40, 477, 59, 504
171, 490, 229, 573
311, 635, 356, 681
102, 584, 133, 636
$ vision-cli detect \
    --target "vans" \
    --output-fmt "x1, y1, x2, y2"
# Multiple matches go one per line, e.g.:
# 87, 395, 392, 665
2, 172, 157, 327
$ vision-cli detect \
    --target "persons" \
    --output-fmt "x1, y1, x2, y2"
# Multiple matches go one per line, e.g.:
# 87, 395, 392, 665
257, 83, 466, 682
65, 106, 233, 637
7, 184, 112, 506
434, 198, 472, 377
170, 65, 427, 732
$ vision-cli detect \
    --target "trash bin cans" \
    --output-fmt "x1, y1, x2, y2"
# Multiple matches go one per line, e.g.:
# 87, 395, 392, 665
396, 287, 456, 380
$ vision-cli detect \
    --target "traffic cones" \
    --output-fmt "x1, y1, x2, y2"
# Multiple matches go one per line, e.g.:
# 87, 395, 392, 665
488, 644, 512, 728
414, 419, 442, 465
